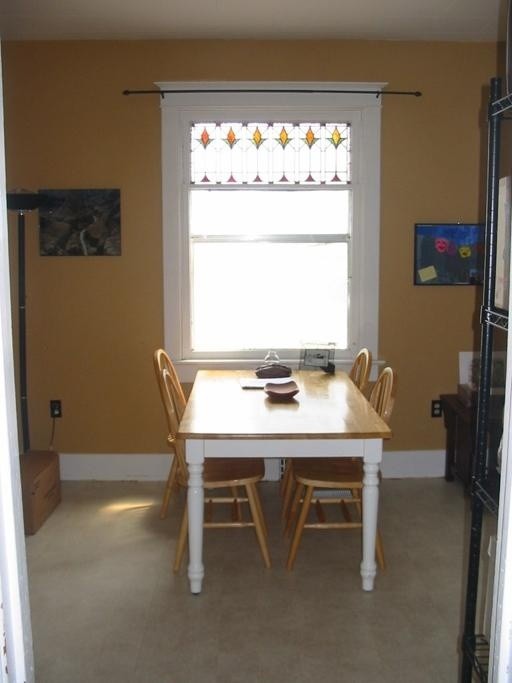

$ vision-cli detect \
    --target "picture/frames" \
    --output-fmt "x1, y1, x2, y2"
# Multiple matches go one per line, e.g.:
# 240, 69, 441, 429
413, 222, 484, 286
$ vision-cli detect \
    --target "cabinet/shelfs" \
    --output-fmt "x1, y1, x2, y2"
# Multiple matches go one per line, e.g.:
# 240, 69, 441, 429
462, 72, 511, 683
438, 393, 505, 494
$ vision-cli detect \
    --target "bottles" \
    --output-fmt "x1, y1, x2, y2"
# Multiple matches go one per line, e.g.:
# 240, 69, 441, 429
265, 350, 280, 366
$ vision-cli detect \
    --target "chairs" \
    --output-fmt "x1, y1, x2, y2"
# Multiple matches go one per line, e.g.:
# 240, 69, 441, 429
159, 368, 272, 571
152, 348, 243, 523
280, 346, 372, 539
282, 366, 395, 571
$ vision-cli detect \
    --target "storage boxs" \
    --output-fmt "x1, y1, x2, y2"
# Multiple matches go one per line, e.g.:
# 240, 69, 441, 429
19, 449, 62, 535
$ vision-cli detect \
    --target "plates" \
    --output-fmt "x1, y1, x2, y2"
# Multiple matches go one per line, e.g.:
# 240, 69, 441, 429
264, 380, 300, 399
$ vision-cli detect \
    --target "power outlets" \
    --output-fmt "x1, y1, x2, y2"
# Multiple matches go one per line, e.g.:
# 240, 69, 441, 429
431, 399, 442, 417
50, 399, 62, 418
